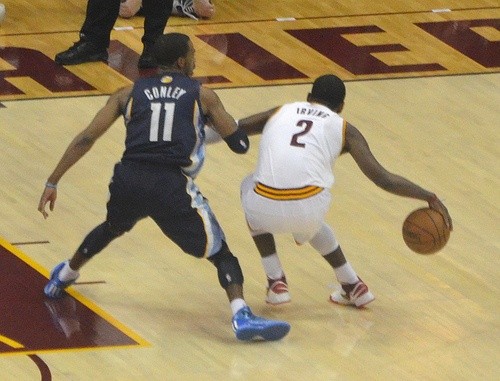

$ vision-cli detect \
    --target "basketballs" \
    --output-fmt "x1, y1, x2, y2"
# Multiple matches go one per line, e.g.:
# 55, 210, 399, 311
402, 207, 450, 256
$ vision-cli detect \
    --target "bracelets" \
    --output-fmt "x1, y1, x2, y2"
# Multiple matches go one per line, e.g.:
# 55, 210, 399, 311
428, 193, 437, 204
46, 182, 57, 189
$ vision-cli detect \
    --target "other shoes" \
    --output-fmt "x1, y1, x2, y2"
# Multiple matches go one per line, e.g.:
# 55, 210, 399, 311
137, 40, 156, 66
57, 41, 108, 63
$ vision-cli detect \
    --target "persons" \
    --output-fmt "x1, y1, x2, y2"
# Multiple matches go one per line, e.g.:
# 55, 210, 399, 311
55, 0, 173, 67
38, 32, 291, 341
205, 73, 453, 308
119, 0, 215, 18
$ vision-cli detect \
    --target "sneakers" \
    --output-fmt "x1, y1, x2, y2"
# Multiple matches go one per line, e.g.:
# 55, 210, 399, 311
331, 282, 373, 309
231, 306, 290, 342
263, 279, 293, 304
45, 263, 74, 297
